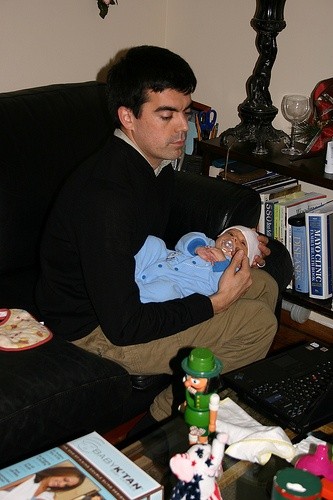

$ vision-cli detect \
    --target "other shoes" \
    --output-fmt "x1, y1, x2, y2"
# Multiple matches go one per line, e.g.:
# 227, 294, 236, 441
124, 413, 159, 445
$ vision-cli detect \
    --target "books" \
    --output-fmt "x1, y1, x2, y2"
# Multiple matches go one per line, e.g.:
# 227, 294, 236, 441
0, 431, 164, 500
208, 156, 333, 300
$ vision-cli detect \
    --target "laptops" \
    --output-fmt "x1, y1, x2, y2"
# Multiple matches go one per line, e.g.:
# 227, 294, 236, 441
217, 338, 333, 434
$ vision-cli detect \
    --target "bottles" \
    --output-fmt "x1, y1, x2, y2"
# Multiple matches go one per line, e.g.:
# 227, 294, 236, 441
271, 444, 333, 500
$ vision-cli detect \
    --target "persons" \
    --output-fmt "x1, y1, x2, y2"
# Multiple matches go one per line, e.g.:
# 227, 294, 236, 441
73, 493, 103, 500
0, 467, 86, 500
133, 226, 263, 304
34, 45, 280, 444
176, 348, 223, 446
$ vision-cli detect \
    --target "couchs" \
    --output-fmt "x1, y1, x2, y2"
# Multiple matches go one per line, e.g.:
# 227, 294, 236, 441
0, 81, 294, 471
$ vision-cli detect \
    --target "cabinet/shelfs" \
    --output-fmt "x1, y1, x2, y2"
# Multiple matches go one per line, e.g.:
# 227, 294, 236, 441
197, 130, 333, 344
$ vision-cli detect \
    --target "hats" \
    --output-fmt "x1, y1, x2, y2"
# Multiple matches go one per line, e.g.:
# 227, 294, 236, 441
217, 225, 261, 268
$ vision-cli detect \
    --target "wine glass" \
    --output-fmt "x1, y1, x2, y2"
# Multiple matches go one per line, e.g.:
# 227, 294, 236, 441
281, 95, 311, 156
